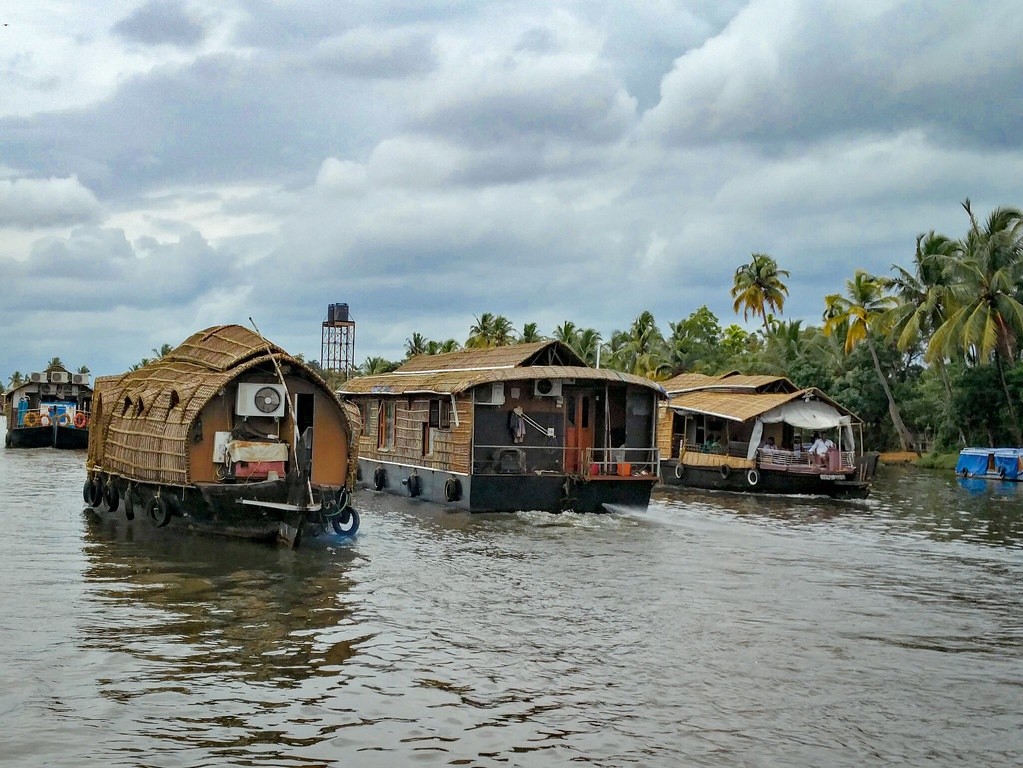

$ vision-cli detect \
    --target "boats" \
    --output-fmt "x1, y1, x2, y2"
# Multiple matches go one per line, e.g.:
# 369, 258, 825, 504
81, 323, 365, 550
652, 368, 882, 500
0, 363, 95, 448
955, 446, 1023, 483
332, 337, 672, 514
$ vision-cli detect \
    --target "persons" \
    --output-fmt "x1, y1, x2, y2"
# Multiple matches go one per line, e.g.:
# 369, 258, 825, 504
703, 434, 714, 452
712, 435, 722, 452
764, 436, 777, 450
808, 431, 833, 463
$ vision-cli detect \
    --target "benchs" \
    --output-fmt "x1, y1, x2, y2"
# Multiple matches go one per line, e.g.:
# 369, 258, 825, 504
729, 440, 765, 457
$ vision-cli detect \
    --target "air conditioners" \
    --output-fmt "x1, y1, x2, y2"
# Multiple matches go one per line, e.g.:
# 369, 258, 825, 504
72, 373, 88, 385
534, 379, 562, 398
30, 372, 48, 384
235, 382, 285, 418
474, 382, 505, 405
51, 371, 68, 384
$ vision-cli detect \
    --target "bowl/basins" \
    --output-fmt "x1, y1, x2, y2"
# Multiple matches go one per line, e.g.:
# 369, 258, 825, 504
617, 464, 631, 476
601, 464, 617, 475
589, 464, 599, 475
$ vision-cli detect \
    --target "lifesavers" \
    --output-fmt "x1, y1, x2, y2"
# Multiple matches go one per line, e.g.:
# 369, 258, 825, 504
675, 463, 684, 479
747, 469, 760, 486
374, 469, 384, 491
961, 467, 967, 477
444, 478, 456, 502
24, 412, 86, 428
83, 477, 171, 529
407, 474, 417, 498
997, 466, 1005, 479
721, 464, 731, 480
332, 505, 360, 535
